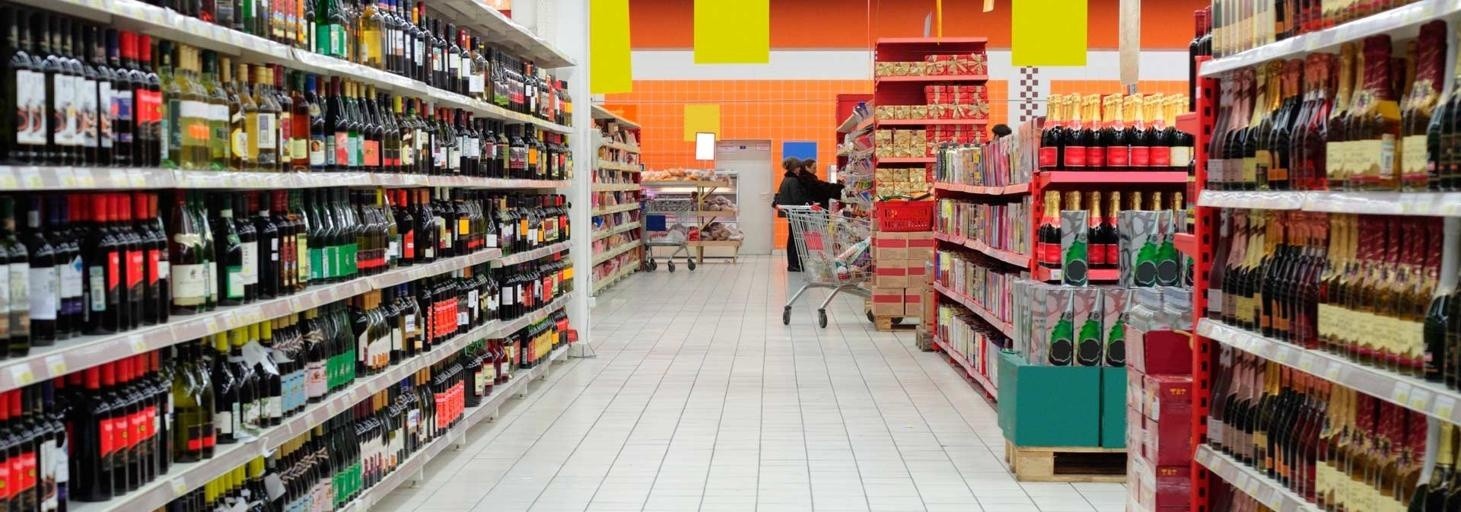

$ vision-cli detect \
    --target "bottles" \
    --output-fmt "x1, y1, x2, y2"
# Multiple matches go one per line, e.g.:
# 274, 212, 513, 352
1157, 213, 1182, 288
1136, 216, 1156, 288
1179, 1, 1413, 109
1038, 86, 1196, 271
935, 137, 988, 185
0, 0, 645, 509
1208, 209, 1460, 393
1047, 292, 1073, 366
1107, 290, 1133, 366
1062, 211, 1089, 285
1205, 14, 1461, 190
1205, 342, 1461, 512
1075, 287, 1104, 367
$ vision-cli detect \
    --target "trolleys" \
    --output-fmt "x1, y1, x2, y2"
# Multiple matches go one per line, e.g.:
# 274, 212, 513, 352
772, 199, 878, 328
639, 187, 698, 270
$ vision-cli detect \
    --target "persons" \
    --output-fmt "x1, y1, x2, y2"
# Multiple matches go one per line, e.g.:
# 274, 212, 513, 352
799, 159, 844, 209
775, 157, 804, 272
991, 124, 1012, 139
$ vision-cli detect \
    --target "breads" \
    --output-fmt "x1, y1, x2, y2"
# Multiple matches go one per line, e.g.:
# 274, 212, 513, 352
642, 168, 729, 181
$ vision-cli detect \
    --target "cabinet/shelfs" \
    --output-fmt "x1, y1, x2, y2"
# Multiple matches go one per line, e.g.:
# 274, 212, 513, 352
930, 169, 1198, 406
637, 169, 742, 265
870, 35, 989, 333
1174, 0, 1461, 512
834, 93, 874, 294
0, 0, 588, 512
590, 103, 648, 299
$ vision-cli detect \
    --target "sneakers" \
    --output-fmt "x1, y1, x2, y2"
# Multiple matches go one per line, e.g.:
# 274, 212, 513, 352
785, 264, 805, 273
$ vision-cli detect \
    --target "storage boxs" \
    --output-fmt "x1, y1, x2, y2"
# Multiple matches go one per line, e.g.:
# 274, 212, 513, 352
870, 202, 934, 317
1123, 323, 1192, 512
994, 277, 1195, 450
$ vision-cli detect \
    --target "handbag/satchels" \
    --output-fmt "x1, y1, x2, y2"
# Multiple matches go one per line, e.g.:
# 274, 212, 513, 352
770, 191, 786, 209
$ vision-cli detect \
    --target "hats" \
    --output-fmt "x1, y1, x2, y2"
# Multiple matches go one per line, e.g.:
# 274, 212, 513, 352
781, 155, 801, 171
